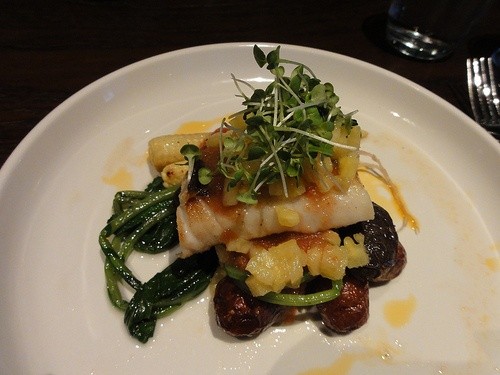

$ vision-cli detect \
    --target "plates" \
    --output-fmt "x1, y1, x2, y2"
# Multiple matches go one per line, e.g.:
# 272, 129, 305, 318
0, 42, 500, 374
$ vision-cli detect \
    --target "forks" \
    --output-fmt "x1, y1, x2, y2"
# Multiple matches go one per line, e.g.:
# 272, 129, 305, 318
466, 57, 500, 142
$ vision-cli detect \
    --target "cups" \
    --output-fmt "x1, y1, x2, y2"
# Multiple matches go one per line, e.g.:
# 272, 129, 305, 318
385, 0, 495, 62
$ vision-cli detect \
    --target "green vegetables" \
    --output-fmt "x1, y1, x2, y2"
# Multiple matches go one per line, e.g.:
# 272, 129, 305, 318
181, 46, 360, 204
98, 176, 342, 343
